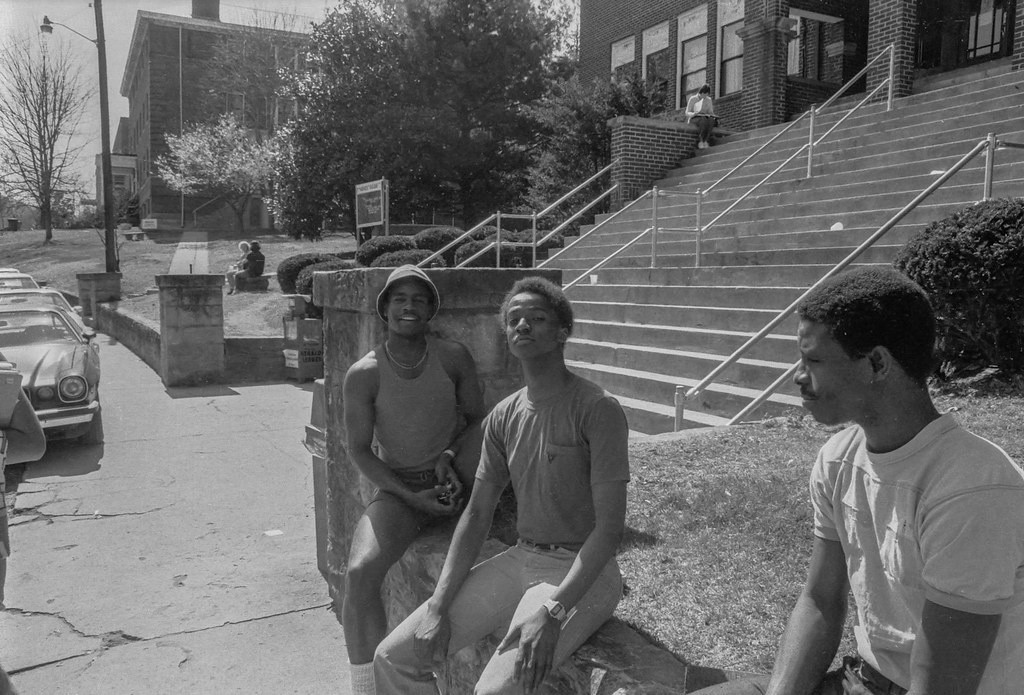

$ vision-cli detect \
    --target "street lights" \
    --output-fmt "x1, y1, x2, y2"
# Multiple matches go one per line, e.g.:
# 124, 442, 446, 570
41, 0, 117, 271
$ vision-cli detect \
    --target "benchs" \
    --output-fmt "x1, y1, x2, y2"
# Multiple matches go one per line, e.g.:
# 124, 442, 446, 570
122, 232, 148, 240
236, 275, 271, 291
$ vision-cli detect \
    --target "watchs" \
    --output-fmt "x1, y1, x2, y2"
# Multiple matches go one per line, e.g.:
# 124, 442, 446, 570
542, 596, 570, 623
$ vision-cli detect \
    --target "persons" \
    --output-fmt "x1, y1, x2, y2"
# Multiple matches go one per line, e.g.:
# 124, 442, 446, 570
367, 273, 632, 695
340, 262, 488, 695
685, 83, 718, 150
671, 267, 1024, 695
232, 240, 265, 294
226, 241, 252, 295
0, 352, 50, 606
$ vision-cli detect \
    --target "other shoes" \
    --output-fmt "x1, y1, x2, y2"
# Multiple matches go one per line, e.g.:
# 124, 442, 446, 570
233, 291, 240, 295
704, 143, 709, 148
228, 291, 232, 295
698, 143, 704, 149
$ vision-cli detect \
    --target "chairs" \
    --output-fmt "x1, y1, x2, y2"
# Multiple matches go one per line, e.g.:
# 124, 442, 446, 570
24, 324, 53, 343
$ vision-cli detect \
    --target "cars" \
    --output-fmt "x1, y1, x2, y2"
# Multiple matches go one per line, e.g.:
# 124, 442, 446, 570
0, 267, 100, 354
0, 304, 103, 447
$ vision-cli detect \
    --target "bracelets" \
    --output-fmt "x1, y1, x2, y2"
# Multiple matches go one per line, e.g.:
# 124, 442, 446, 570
442, 450, 457, 459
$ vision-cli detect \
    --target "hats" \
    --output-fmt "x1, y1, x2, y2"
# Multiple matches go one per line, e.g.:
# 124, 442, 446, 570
376, 264, 441, 324
